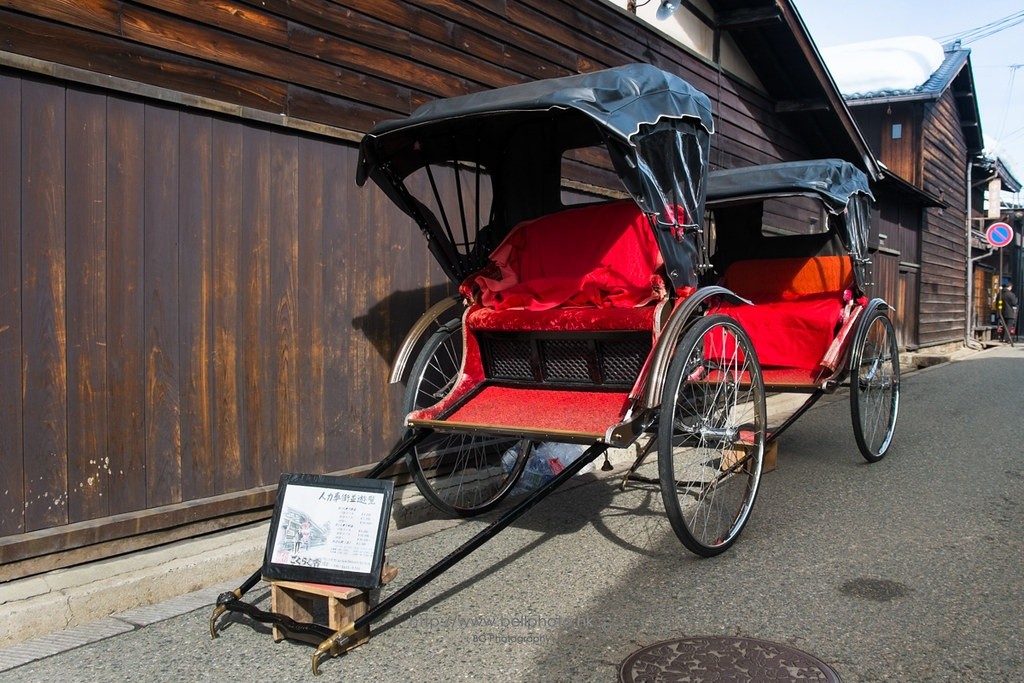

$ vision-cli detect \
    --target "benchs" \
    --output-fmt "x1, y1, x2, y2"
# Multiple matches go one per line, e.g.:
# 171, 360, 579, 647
701, 256, 854, 369
460, 196, 686, 337
974, 323, 997, 341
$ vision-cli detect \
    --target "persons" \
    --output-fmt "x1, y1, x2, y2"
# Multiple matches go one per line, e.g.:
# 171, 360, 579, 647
995, 282, 1019, 342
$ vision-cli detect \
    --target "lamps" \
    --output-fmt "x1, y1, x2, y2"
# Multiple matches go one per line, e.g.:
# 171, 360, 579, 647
627, 0, 681, 22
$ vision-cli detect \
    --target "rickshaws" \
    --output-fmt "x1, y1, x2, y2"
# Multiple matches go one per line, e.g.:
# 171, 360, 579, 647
619, 157, 903, 502
206, 60, 769, 679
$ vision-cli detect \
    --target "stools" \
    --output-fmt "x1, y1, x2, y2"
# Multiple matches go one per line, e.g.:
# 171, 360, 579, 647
261, 564, 399, 656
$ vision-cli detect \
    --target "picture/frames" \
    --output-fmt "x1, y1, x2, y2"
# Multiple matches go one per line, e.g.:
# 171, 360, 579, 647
261, 472, 397, 590
